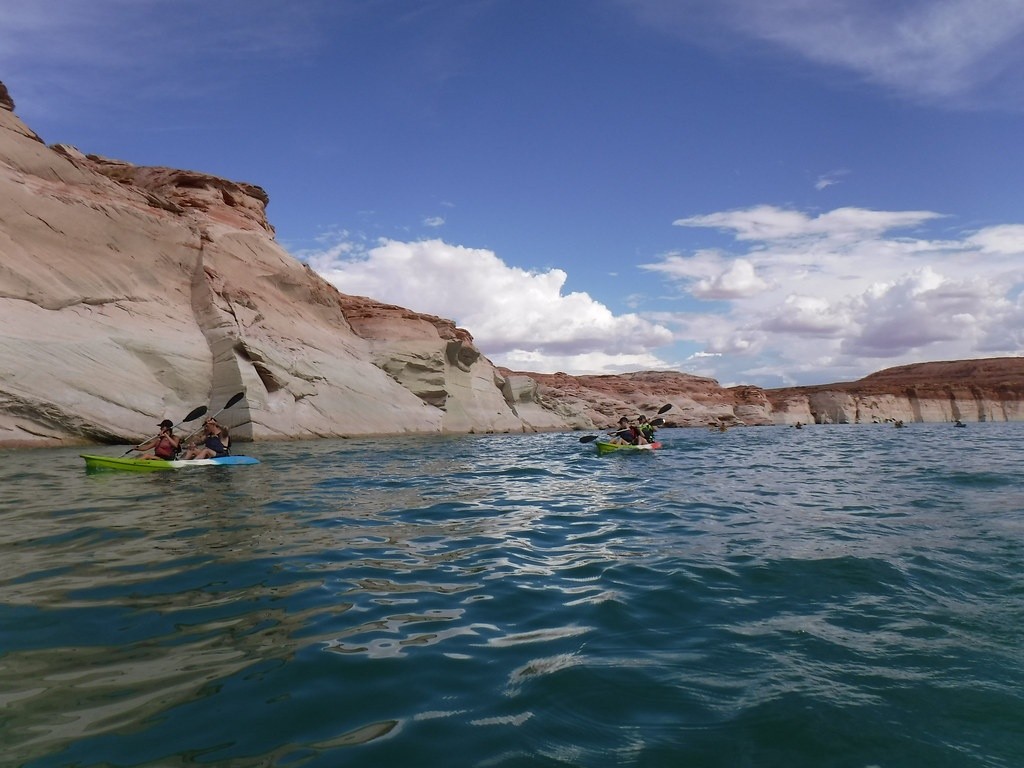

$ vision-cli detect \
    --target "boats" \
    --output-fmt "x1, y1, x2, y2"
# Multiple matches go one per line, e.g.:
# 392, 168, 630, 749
79, 455, 261, 472
596, 442, 662, 455
954, 424, 966, 427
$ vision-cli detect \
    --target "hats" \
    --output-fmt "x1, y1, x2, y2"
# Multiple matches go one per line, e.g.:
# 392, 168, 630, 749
157, 420, 173, 427
637, 415, 646, 419
617, 417, 628, 423
202, 418, 217, 426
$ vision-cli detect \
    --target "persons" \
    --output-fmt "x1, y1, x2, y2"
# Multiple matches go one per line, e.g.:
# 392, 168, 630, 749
181, 418, 230, 460
797, 422, 800, 426
610, 417, 637, 445
895, 419, 904, 425
632, 415, 654, 445
135, 420, 180, 461
957, 421, 961, 425
721, 423, 726, 430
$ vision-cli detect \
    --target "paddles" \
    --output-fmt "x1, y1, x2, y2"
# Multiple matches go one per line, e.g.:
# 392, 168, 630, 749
117, 405, 208, 459
580, 418, 663, 444
638, 403, 672, 427
181, 391, 245, 443
